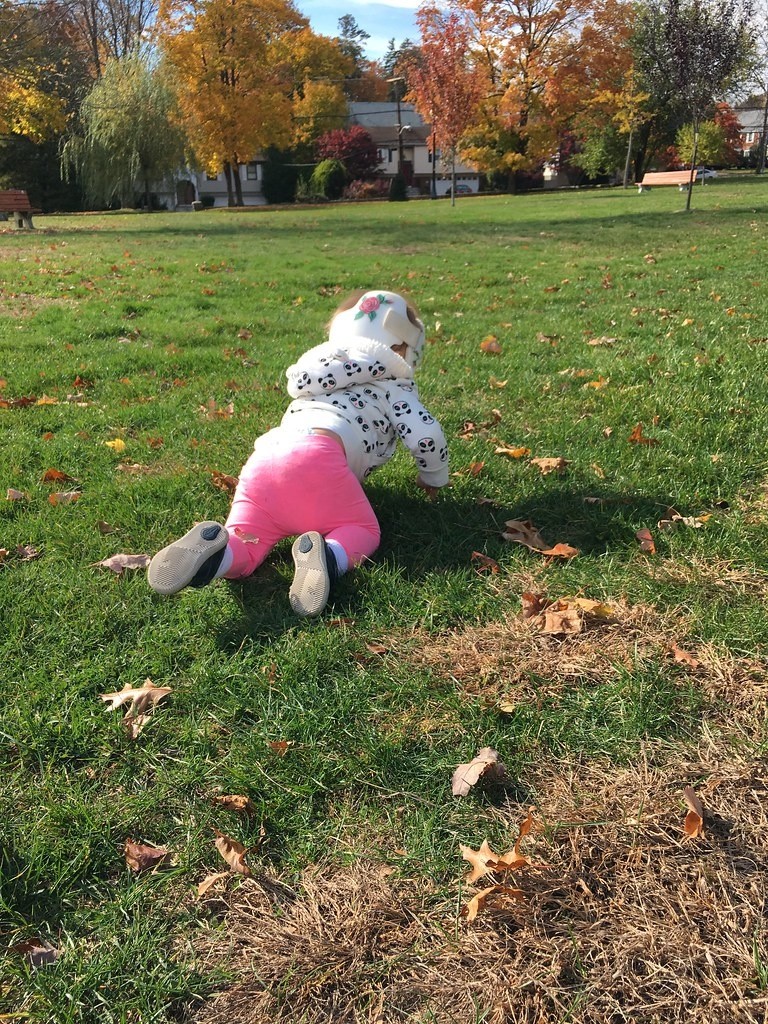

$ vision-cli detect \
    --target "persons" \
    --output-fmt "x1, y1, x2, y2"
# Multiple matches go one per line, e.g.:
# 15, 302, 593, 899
146, 288, 452, 617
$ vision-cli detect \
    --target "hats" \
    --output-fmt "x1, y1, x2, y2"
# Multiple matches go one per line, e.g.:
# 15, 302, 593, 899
329, 289, 425, 367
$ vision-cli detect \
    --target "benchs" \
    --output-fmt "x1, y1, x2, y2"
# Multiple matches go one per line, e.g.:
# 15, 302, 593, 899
0, 190, 42, 230
634, 169, 697, 194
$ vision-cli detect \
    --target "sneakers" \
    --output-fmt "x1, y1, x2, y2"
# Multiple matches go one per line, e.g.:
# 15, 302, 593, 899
289, 530, 342, 619
148, 521, 229, 596
